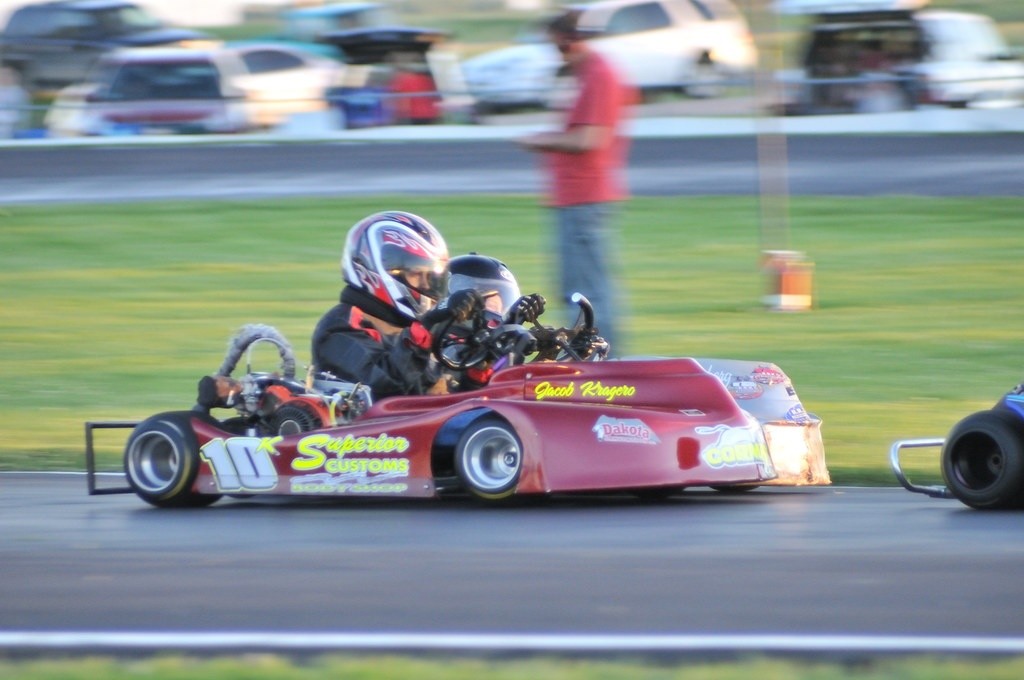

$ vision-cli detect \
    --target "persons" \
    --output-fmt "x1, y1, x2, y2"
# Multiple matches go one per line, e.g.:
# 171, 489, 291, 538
448, 253, 610, 392
310, 210, 485, 405
519, 16, 621, 359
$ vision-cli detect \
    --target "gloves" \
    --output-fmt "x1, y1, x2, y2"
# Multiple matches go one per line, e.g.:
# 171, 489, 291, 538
422, 288, 485, 323
506, 293, 548, 323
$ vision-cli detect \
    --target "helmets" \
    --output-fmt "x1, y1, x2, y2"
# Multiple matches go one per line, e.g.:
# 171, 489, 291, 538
442, 252, 521, 329
342, 211, 449, 319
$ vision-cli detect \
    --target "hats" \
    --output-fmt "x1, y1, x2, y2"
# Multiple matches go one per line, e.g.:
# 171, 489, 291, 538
546, 9, 599, 38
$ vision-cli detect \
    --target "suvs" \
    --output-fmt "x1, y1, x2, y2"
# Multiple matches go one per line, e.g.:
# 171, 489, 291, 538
1, 0, 1024, 133
